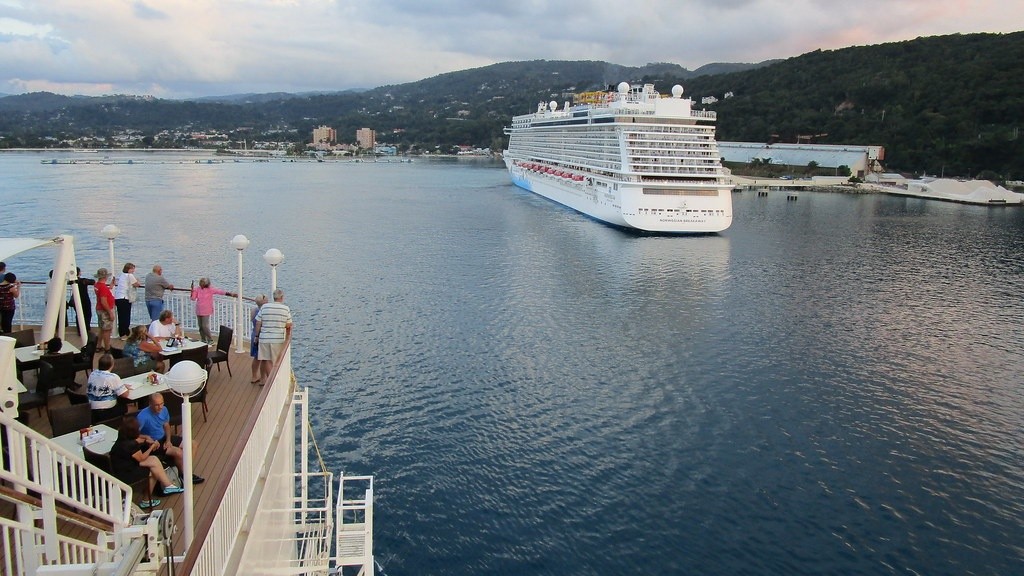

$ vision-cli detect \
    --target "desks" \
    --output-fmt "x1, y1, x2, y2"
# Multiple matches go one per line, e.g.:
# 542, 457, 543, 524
149, 337, 208, 357
117, 371, 170, 401
83, 423, 119, 475
15, 341, 82, 376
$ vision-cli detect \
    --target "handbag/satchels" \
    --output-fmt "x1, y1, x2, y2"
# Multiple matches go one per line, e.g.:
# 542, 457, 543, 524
128, 274, 139, 303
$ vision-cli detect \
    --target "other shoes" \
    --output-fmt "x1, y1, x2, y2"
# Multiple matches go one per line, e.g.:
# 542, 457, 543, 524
140, 499, 161, 508
193, 474, 205, 483
208, 340, 215, 346
163, 486, 185, 494
71, 382, 82, 392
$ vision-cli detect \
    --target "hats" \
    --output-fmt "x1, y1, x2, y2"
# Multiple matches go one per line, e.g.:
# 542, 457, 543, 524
94, 268, 112, 279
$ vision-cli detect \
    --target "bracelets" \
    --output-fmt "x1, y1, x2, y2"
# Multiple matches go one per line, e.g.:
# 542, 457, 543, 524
175, 324, 179, 325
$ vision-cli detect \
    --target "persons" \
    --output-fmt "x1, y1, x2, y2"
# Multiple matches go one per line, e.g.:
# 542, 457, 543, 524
43, 337, 82, 392
146, 310, 181, 341
191, 277, 237, 347
145, 264, 174, 321
0, 261, 21, 335
114, 263, 140, 341
69, 267, 95, 336
45, 270, 69, 337
109, 392, 205, 508
85, 354, 132, 426
93, 268, 116, 354
122, 324, 165, 374
250, 288, 293, 387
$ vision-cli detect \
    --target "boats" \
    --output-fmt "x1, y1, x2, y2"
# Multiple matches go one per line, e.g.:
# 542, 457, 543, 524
515, 161, 543, 173
543, 168, 556, 179
536, 166, 548, 176
553, 170, 565, 180
571, 174, 585, 185
561, 172, 572, 183
0, 234, 375, 576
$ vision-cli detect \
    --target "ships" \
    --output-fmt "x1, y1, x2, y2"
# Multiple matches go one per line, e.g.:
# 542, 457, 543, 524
500, 82, 735, 236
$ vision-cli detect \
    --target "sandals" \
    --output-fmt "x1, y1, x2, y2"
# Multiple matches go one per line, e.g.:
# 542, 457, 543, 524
105, 345, 114, 354
96, 347, 105, 352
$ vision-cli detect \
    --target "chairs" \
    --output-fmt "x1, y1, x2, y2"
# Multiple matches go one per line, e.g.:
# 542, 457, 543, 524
6, 325, 234, 511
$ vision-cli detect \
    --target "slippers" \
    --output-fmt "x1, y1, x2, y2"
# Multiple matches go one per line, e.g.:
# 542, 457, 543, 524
251, 378, 260, 383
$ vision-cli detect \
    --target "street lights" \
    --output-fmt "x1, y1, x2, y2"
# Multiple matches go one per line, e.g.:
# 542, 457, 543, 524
161, 361, 207, 554
229, 233, 250, 354
263, 248, 285, 303
101, 225, 120, 281
